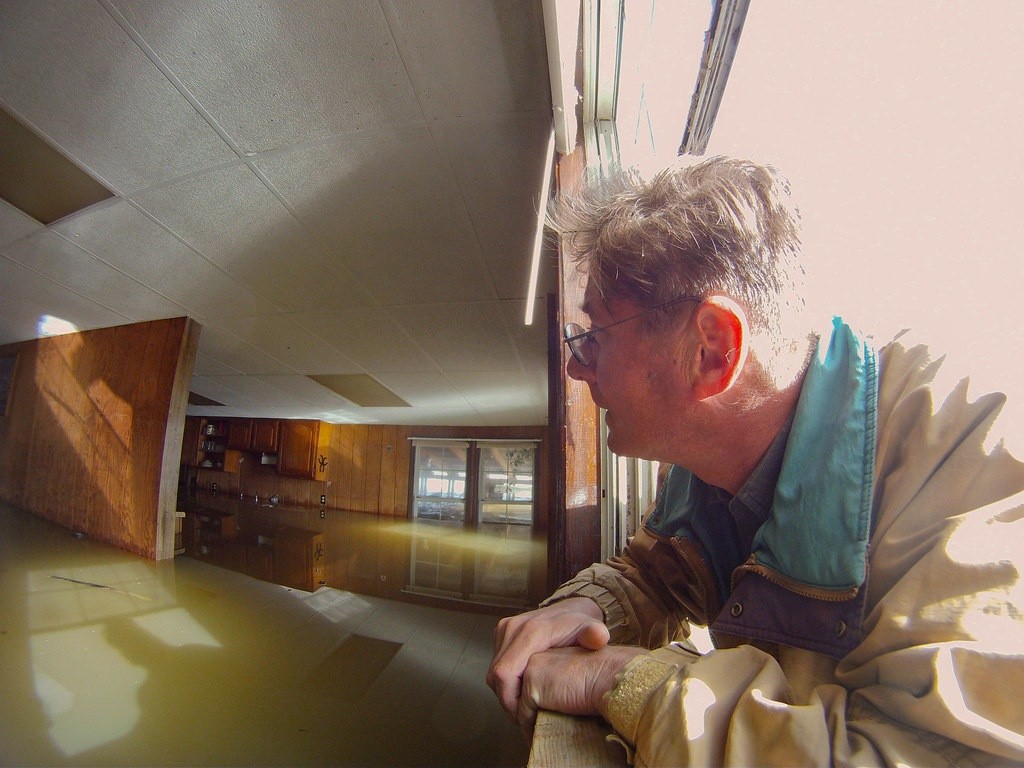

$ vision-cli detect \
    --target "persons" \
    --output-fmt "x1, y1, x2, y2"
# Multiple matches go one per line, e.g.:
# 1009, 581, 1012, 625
486, 156, 1024, 768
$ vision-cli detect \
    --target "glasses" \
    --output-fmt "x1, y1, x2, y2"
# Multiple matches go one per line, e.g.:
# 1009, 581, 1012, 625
564, 296, 702, 365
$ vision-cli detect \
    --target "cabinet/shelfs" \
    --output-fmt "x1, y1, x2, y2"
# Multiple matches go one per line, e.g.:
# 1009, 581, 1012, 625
181, 417, 330, 482
184, 508, 325, 593
